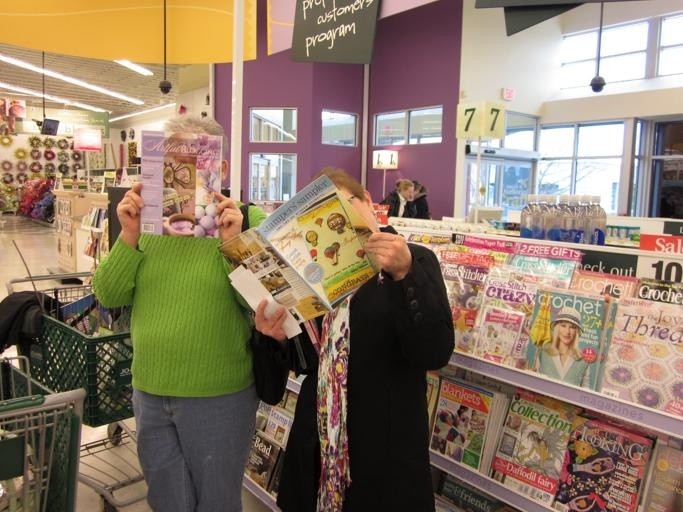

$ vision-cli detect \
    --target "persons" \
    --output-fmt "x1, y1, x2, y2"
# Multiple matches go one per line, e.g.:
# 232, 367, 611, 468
90, 117, 266, 512
412, 179, 429, 219
377, 178, 418, 217
253, 164, 456, 511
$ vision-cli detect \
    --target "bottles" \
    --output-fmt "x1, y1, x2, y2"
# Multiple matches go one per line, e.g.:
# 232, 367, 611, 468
520, 195, 606, 246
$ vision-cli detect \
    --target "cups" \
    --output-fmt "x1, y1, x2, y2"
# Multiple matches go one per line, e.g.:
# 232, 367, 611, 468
72, 185, 78, 190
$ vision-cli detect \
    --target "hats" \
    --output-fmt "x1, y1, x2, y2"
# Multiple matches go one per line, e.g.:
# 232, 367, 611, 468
550, 307, 584, 338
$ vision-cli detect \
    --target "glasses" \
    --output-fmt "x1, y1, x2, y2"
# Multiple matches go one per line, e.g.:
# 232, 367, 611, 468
347, 195, 362, 204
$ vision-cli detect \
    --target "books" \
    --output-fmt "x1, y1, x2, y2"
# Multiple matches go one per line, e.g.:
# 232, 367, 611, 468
403, 232, 683, 421
424, 365, 683, 512
425, 370, 683, 512
217, 173, 383, 324
242, 390, 299, 500
137, 129, 223, 238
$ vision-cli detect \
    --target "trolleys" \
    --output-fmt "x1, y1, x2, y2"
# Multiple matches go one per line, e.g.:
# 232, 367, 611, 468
1, 356, 83, 511
5, 272, 148, 510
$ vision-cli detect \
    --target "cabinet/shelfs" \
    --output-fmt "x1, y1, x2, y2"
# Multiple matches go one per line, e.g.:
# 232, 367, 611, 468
242, 206, 683, 512
